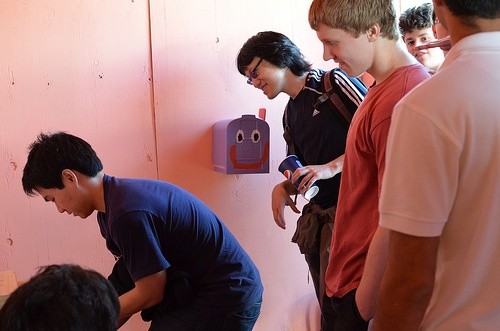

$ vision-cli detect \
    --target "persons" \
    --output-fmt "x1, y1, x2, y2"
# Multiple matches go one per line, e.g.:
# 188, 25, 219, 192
237, 31, 369, 310
22, 133, 264, 331
399, 2, 453, 75
368, 0, 500, 331
308, 0, 433, 331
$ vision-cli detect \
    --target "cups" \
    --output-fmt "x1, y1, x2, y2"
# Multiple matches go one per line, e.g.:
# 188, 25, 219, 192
278, 155, 321, 199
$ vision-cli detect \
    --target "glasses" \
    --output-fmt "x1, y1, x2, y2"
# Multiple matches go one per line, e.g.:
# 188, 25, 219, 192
247, 56, 264, 85
